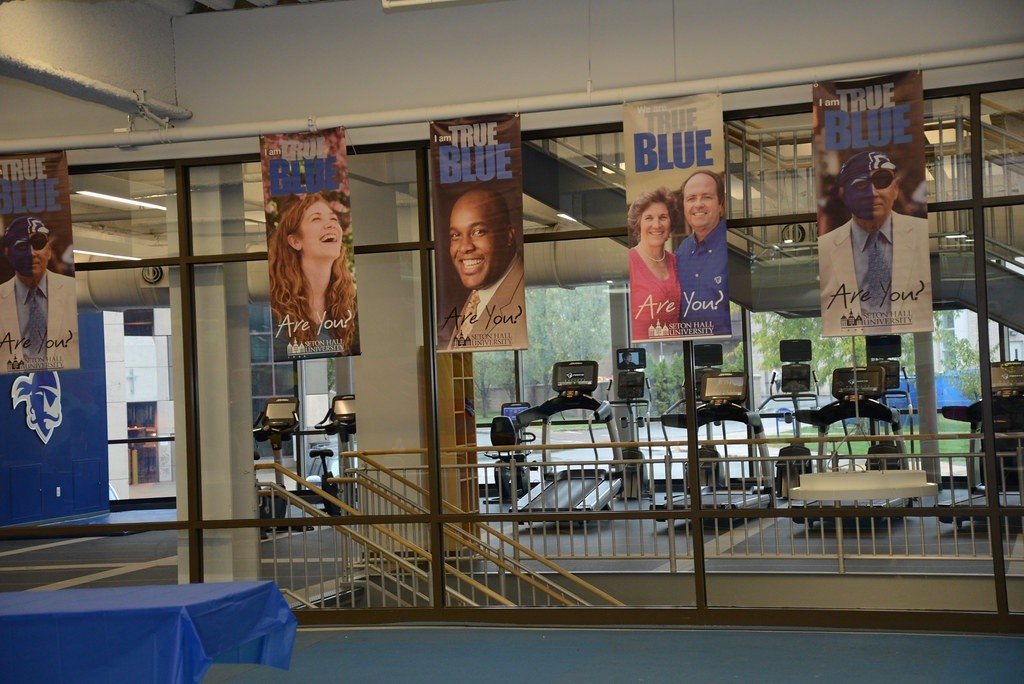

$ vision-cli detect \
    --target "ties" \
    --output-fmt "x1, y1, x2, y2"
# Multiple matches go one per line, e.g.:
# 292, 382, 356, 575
453, 290, 481, 348
866, 231, 892, 306
28, 286, 47, 360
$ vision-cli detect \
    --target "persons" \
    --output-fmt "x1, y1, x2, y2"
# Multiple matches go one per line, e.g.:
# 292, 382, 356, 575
439, 183, 526, 350
619, 353, 636, 366
0, 217, 80, 370
270, 193, 358, 357
819, 151, 931, 333
628, 172, 730, 340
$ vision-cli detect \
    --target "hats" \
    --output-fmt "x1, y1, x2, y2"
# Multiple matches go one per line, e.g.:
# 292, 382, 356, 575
3, 215, 50, 247
836, 151, 896, 189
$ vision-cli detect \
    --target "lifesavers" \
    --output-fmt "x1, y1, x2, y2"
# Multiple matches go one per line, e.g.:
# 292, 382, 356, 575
782, 223, 806, 242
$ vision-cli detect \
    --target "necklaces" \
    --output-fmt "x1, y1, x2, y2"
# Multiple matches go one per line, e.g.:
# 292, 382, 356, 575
638, 247, 665, 263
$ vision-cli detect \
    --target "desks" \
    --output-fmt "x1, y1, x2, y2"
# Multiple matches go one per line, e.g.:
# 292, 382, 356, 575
0, 580, 298, 684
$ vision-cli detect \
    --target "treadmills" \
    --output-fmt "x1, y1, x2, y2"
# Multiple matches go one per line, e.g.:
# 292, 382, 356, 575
651, 373, 775, 517
510, 361, 630, 521
939, 361, 1023, 522
786, 367, 912, 519
